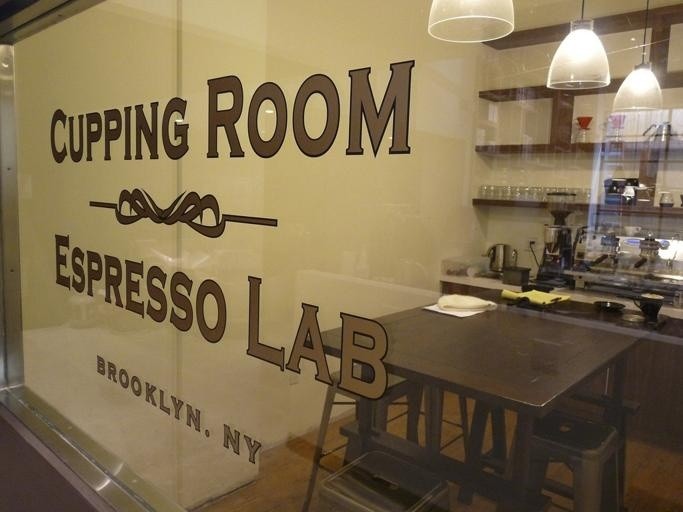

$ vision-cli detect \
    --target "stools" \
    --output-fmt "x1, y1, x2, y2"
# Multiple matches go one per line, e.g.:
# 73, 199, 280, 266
301, 363, 471, 510
509, 411, 627, 512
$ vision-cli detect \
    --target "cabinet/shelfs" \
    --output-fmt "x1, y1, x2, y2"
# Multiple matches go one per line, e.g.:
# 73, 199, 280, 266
466, 0, 683, 220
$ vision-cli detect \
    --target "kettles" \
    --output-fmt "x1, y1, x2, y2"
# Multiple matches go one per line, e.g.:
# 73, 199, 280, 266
485, 243, 518, 273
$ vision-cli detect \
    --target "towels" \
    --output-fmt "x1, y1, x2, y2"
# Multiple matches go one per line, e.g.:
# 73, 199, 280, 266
437, 292, 498, 313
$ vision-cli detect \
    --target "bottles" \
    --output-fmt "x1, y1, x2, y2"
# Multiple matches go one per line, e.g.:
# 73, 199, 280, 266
659, 192, 674, 207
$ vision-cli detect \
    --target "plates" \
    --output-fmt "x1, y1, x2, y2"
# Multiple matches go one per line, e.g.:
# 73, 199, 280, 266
592, 301, 624, 312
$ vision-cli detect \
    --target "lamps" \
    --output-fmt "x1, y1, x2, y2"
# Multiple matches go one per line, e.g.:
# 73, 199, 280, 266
425, 0, 666, 115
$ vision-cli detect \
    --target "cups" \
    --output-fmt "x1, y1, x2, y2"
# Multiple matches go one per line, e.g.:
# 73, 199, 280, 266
623, 225, 641, 237
478, 184, 591, 205
633, 291, 665, 320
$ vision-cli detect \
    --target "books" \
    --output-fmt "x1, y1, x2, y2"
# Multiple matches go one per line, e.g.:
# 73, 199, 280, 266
501, 289, 570, 305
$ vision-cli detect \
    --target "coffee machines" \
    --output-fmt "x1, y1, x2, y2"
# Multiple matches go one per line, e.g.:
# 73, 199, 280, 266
534, 191, 575, 287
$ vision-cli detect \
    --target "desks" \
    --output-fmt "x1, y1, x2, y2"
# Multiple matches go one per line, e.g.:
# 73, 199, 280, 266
293, 297, 648, 509
